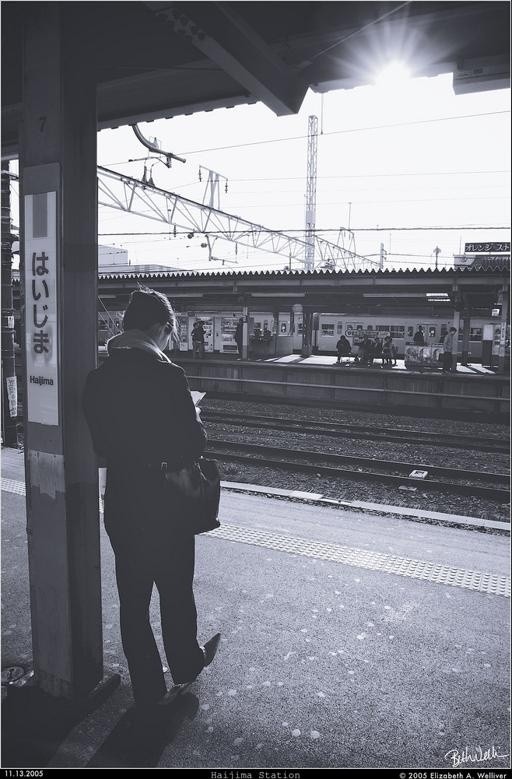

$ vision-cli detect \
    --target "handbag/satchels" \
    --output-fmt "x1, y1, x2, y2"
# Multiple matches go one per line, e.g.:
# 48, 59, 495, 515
158, 361, 221, 536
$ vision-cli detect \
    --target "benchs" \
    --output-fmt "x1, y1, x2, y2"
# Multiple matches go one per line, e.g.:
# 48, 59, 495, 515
334, 345, 399, 367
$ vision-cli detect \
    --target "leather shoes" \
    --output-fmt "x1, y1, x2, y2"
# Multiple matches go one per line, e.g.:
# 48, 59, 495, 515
135, 686, 180, 711
204, 632, 220, 668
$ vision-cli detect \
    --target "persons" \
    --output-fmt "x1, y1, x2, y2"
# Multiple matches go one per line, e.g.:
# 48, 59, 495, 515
82, 291, 221, 706
192, 321, 206, 359
336, 326, 456, 373
234, 318, 243, 360
